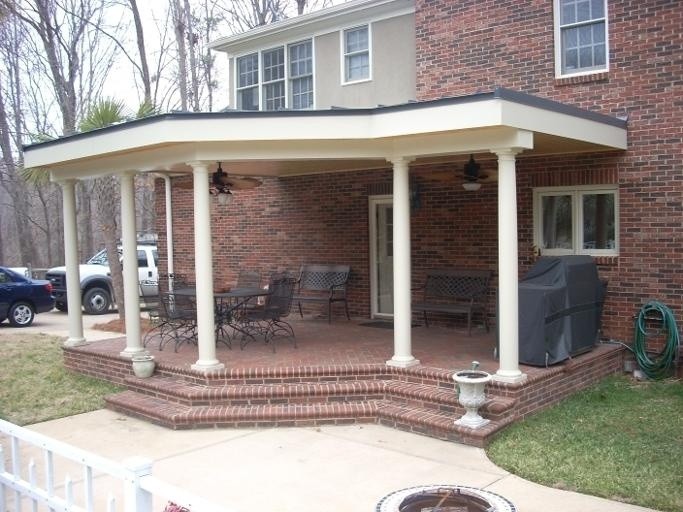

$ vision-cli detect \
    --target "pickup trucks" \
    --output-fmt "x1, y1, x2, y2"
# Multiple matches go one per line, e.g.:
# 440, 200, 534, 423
45, 239, 159, 314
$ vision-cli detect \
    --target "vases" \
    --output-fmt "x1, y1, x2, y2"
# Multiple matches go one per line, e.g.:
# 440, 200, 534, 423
452, 370, 492, 429
131, 356, 155, 378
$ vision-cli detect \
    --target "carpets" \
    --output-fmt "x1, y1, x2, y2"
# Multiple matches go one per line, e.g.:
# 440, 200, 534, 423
358, 320, 423, 329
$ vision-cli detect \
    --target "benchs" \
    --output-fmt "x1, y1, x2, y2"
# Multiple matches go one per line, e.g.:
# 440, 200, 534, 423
292, 264, 352, 325
412, 269, 495, 337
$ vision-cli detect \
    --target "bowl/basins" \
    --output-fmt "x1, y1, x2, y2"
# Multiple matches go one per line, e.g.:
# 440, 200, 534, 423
213, 287, 231, 293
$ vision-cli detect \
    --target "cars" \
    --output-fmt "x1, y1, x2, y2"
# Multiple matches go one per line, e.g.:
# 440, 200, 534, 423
0, 266, 56, 328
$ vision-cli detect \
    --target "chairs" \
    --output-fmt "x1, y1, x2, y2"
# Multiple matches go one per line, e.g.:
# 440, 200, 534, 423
139, 271, 296, 352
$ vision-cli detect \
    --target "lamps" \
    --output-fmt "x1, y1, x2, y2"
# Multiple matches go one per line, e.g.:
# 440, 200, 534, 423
460, 154, 488, 190
209, 161, 233, 205
